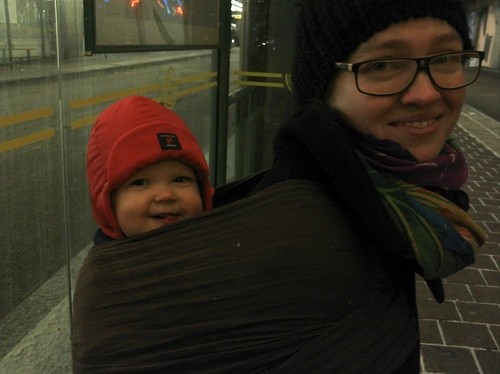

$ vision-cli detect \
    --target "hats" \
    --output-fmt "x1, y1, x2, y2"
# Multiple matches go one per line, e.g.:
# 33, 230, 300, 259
292, 0, 473, 104
85, 95, 209, 241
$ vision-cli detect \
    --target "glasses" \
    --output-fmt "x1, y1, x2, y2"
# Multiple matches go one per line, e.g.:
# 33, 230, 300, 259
331, 49, 486, 98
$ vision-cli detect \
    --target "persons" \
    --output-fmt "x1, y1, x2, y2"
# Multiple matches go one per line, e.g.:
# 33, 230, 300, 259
72, 0, 491, 374
84, 95, 215, 248
